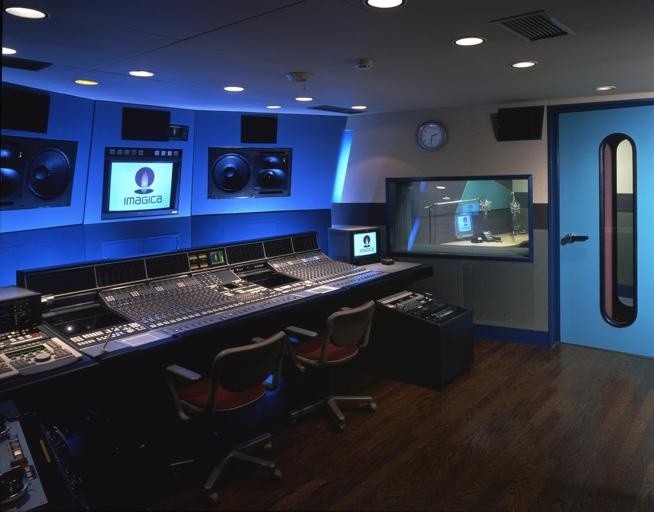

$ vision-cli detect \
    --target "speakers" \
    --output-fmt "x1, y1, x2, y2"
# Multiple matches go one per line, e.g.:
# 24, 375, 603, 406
490, 105, 545, 142
122, 106, 171, 142
0, 136, 78, 210
1, 82, 51, 134
241, 115, 277, 144
208, 147, 291, 198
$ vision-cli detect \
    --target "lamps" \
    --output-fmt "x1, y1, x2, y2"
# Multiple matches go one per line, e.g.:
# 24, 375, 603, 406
285, 73, 315, 84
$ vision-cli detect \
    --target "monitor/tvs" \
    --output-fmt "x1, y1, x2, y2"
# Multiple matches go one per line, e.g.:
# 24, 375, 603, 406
328, 224, 381, 264
101, 146, 183, 220
453, 213, 474, 239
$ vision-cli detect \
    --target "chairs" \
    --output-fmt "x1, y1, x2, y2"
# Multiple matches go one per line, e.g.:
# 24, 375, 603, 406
287, 296, 380, 434
177, 331, 287, 505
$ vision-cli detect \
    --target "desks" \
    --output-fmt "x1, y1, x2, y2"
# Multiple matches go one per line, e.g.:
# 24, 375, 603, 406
439, 230, 528, 247
0, 260, 434, 395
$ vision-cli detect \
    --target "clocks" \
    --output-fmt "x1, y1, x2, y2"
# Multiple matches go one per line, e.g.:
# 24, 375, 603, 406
417, 120, 448, 151
